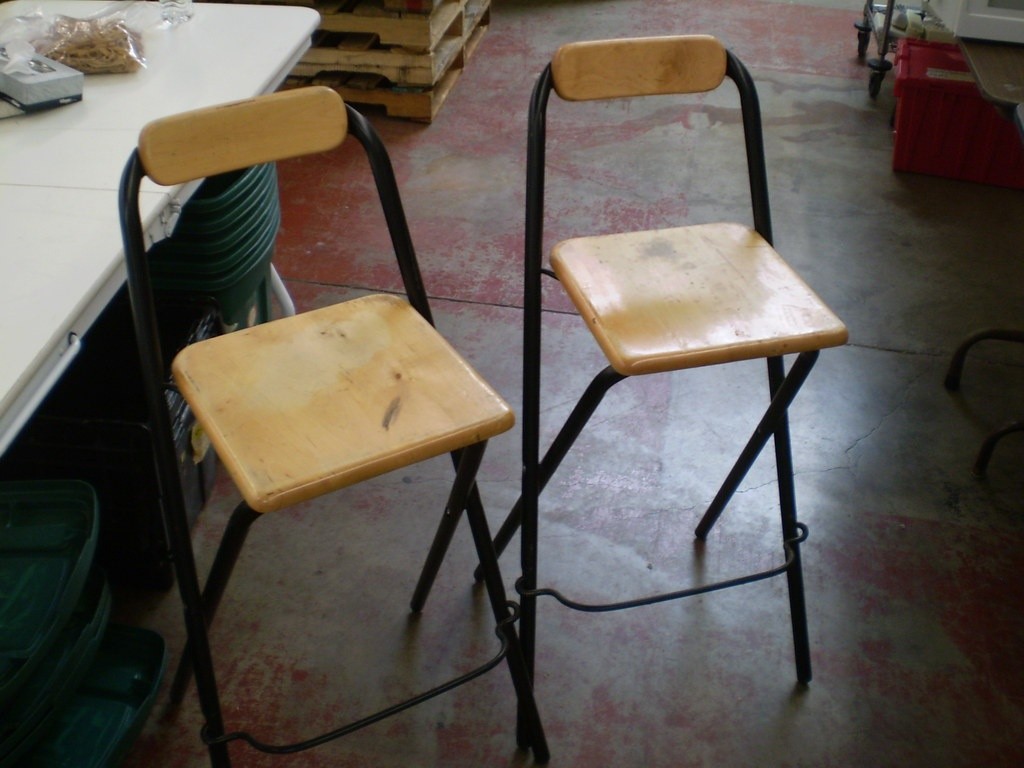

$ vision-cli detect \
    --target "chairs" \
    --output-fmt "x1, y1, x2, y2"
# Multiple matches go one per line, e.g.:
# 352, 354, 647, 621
473, 33, 851, 750
117, 89, 553, 768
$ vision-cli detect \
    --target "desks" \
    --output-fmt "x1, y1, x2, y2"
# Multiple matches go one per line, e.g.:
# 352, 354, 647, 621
921, 1, 1024, 108
0, 0, 320, 460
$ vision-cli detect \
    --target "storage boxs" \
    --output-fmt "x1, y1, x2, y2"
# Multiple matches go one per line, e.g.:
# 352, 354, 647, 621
0, 45, 84, 112
893, 38, 1024, 189
1, 289, 225, 596
